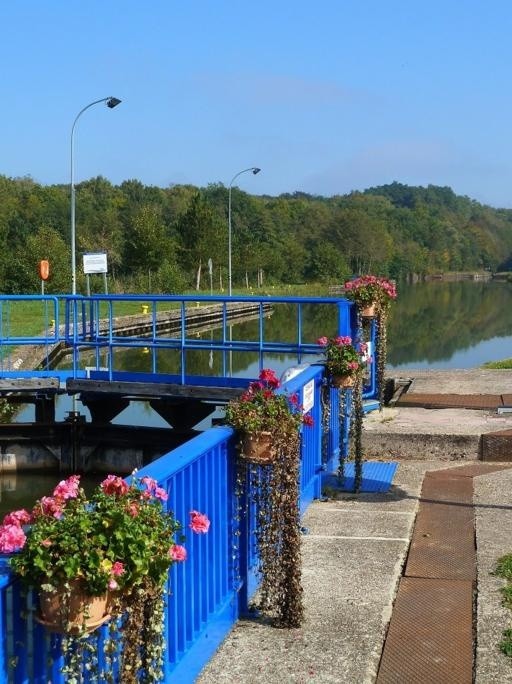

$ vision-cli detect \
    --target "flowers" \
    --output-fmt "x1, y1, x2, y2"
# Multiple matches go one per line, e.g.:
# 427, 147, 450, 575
0, 465, 209, 684
217, 366, 316, 629
317, 335, 377, 504
343, 274, 397, 414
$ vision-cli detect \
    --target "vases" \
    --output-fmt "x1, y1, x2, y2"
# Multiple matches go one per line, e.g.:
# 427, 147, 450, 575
37, 567, 107, 635
359, 299, 377, 317
332, 370, 352, 385
242, 428, 275, 463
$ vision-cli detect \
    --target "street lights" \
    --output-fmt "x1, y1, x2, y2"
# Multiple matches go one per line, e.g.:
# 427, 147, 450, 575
68, 93, 125, 303
227, 165, 265, 298
